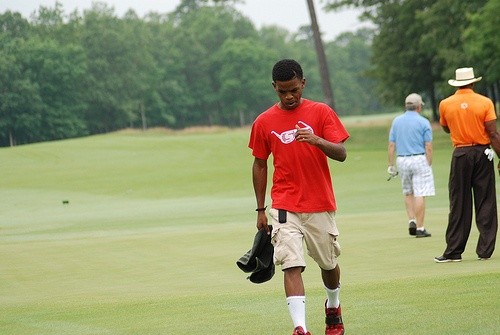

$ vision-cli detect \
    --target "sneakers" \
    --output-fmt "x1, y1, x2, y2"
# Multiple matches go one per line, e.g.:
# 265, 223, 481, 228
324, 299, 345, 335
477, 254, 490, 260
292, 326, 311, 335
433, 255, 462, 262
409, 221, 416, 235
416, 228, 431, 237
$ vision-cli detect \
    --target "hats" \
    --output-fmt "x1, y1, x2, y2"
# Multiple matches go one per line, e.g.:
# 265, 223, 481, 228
405, 93, 424, 106
448, 67, 482, 86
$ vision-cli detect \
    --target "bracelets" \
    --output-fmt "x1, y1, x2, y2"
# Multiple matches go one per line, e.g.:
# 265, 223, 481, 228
255, 206, 267, 211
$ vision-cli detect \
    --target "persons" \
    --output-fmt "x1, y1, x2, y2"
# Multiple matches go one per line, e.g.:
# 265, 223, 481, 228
387, 93, 435, 238
434, 67, 500, 263
247, 60, 351, 335
